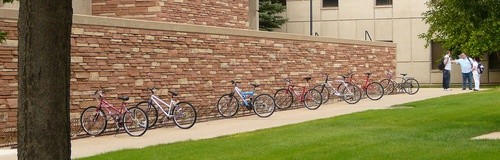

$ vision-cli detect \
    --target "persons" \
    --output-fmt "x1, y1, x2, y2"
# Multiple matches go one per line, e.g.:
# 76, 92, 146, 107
451, 53, 474, 90
471, 57, 480, 91
443, 51, 451, 91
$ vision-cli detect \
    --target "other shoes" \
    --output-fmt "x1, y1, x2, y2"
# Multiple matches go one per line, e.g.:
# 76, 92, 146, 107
469, 87, 472, 90
462, 88, 466, 90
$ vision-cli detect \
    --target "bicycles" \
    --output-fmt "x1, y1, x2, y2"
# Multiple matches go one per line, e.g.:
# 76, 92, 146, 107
273, 76, 322, 110
80, 89, 148, 137
133, 85, 197, 129
311, 71, 384, 105
380, 71, 419, 96
217, 80, 276, 118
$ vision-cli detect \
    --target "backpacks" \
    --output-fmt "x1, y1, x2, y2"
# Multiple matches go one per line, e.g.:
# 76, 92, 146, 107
477, 64, 484, 74
438, 60, 445, 70
